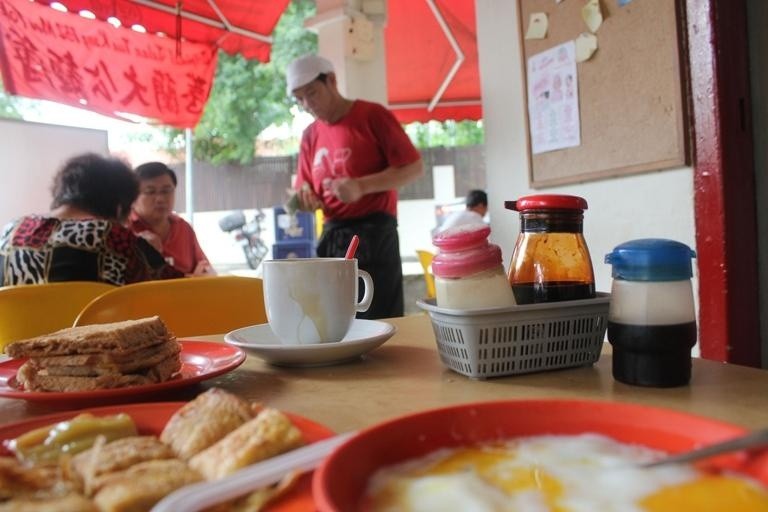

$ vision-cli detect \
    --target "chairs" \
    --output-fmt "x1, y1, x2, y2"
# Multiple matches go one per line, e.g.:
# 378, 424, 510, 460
74, 277, 269, 342
412, 245, 441, 301
1, 280, 119, 356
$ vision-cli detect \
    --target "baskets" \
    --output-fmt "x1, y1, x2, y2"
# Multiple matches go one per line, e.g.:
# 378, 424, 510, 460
414, 290, 612, 383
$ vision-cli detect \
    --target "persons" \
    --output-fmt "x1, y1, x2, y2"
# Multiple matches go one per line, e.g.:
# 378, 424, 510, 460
123, 161, 217, 278
1, 149, 185, 286
441, 189, 488, 237
285, 53, 424, 319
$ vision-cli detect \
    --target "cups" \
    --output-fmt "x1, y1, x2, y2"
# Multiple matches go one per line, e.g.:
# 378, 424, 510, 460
262, 255, 375, 345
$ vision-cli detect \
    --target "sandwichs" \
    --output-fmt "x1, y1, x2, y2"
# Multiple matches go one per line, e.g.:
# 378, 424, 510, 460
4, 315, 183, 393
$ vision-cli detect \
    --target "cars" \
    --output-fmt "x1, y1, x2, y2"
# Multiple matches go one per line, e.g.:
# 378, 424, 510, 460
430, 193, 490, 243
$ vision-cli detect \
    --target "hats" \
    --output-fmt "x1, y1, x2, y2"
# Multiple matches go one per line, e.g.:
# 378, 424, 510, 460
285, 53, 335, 98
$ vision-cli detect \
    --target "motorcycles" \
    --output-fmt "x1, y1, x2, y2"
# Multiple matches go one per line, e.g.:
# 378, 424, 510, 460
218, 202, 269, 268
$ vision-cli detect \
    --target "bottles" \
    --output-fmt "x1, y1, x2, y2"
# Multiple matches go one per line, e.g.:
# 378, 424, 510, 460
601, 237, 700, 389
429, 223, 517, 311
499, 193, 595, 304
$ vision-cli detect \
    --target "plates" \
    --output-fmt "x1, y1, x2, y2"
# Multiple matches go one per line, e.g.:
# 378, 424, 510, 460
0, 338, 248, 404
308, 396, 768, 512
222, 322, 394, 369
1, 400, 337, 512
